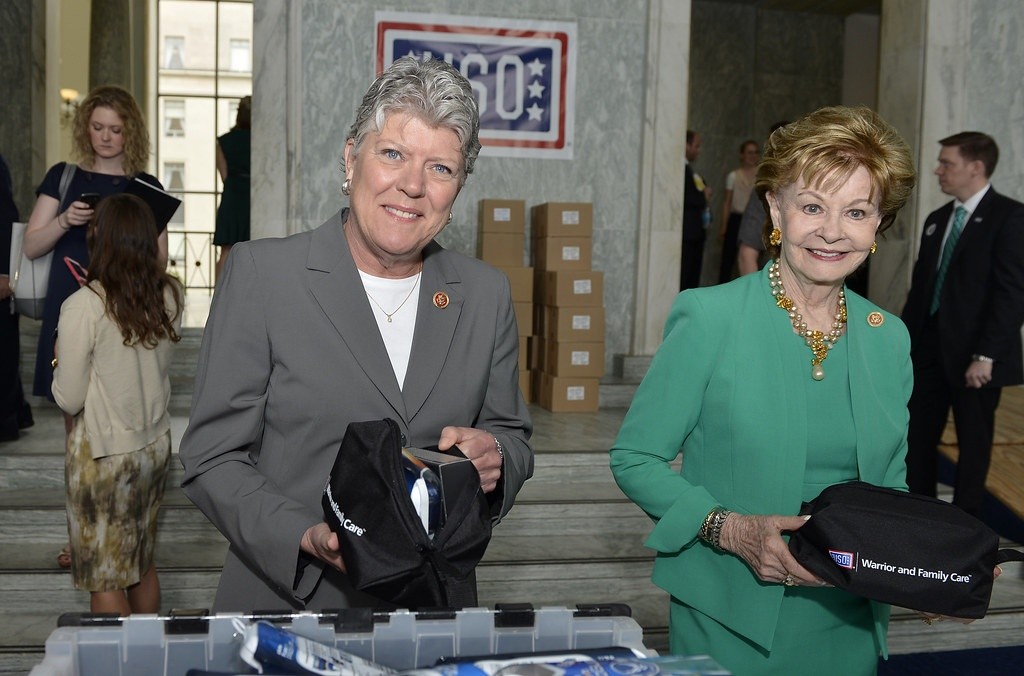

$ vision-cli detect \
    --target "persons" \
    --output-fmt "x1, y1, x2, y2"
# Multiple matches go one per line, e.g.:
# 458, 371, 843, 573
737, 122, 793, 278
902, 133, 1024, 517
210, 97, 251, 291
0, 153, 36, 443
608, 107, 1003, 676
51, 193, 186, 622
719, 140, 768, 285
680, 130, 713, 291
25, 85, 169, 567
176, 59, 535, 617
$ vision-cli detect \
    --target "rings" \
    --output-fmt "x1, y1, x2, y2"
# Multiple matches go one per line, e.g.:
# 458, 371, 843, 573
939, 614, 944, 622
781, 575, 795, 587
921, 617, 932, 626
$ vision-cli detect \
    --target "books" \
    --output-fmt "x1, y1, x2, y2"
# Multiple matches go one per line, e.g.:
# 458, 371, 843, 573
129, 178, 182, 240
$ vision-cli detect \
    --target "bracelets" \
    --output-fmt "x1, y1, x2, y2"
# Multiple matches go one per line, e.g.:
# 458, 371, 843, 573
57, 215, 70, 229
971, 353, 993, 364
699, 505, 731, 553
51, 358, 58, 367
494, 436, 503, 460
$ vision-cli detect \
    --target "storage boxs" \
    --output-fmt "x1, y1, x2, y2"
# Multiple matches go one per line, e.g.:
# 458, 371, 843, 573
478, 199, 605, 412
29, 601, 660, 675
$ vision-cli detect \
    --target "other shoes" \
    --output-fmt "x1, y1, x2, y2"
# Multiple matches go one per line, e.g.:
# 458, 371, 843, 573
0, 412, 35, 444
57, 548, 72, 567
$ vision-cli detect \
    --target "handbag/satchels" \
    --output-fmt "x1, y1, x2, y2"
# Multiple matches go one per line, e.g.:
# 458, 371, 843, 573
9, 162, 77, 320
324, 419, 493, 594
788, 480, 1001, 619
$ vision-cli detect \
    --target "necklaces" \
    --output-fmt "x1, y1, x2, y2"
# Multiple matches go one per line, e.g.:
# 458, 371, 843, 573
365, 261, 422, 322
767, 258, 847, 381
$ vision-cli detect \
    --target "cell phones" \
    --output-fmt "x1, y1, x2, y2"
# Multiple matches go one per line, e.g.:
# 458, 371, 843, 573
80, 192, 101, 210
64, 255, 88, 285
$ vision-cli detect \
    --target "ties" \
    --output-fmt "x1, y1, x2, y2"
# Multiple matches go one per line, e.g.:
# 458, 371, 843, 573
929, 205, 967, 315
